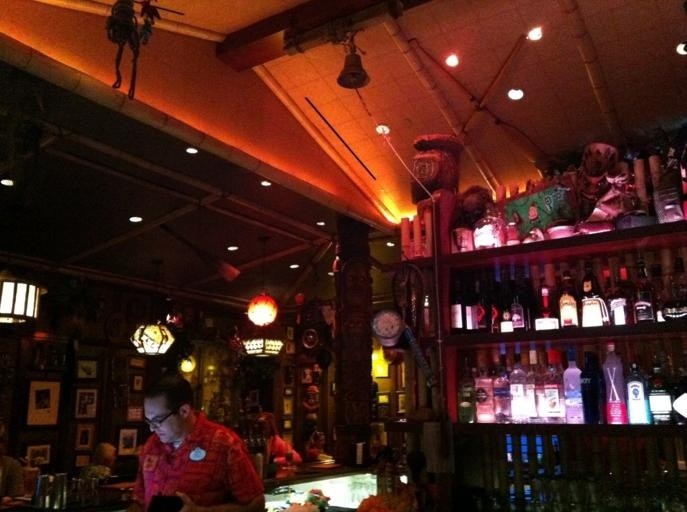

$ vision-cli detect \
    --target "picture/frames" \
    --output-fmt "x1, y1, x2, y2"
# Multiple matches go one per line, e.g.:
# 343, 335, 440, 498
24, 378, 60, 428
26, 442, 51, 465
126, 405, 144, 422
73, 424, 95, 452
133, 374, 145, 392
117, 428, 137, 457
73, 389, 98, 419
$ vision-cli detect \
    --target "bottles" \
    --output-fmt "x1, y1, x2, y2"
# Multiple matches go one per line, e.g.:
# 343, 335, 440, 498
633, 257, 655, 325
625, 363, 651, 424
648, 340, 672, 422
448, 267, 475, 336
663, 258, 687, 321
557, 269, 580, 328
563, 351, 585, 425
494, 367, 510, 424
580, 260, 611, 326
649, 263, 670, 324
526, 351, 545, 424
542, 350, 567, 423
475, 367, 493, 424
601, 342, 628, 425
510, 352, 526, 423
478, 260, 557, 331
615, 266, 638, 325
579, 345, 607, 425
457, 357, 474, 423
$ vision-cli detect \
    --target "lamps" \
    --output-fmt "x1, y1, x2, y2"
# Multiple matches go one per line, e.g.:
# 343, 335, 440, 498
243, 339, 285, 357
130, 323, 176, 356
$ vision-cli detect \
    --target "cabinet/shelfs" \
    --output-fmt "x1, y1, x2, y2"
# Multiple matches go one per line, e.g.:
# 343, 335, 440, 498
436, 190, 687, 429
107, 463, 380, 512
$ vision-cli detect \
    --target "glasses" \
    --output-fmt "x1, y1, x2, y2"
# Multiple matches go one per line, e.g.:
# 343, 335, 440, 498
142, 407, 184, 428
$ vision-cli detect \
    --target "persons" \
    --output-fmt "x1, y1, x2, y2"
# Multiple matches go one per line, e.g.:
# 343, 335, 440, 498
305, 431, 325, 462
126, 375, 266, 512
